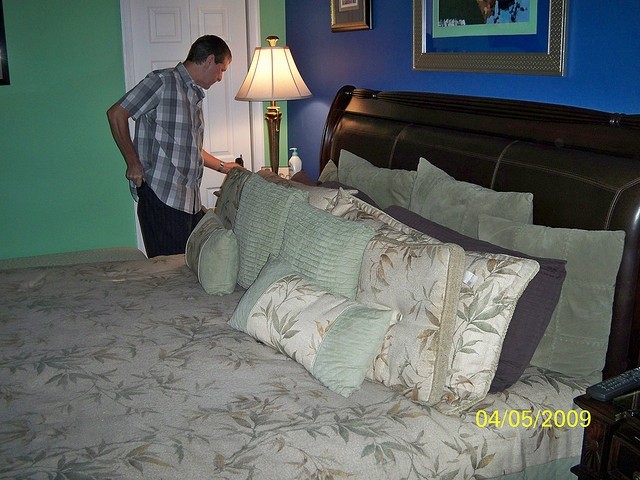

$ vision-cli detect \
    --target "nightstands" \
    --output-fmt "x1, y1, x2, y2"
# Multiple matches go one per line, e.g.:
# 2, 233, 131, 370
569, 387, 640, 480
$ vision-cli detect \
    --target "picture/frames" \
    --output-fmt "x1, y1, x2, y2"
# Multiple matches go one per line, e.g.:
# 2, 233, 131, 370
330, 0, 373, 33
412, 0, 568, 77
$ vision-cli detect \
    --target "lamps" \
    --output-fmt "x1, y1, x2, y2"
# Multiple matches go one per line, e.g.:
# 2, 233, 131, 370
235, 35, 313, 175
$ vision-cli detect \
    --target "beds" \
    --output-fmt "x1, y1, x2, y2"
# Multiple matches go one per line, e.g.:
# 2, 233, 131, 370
1, 85, 638, 480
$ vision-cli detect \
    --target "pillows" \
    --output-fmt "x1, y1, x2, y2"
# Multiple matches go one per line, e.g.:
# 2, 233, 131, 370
356, 227, 465, 407
409, 156, 533, 239
184, 208, 240, 295
328, 185, 540, 417
317, 158, 337, 184
291, 170, 380, 210
380, 205, 566, 394
234, 173, 308, 291
228, 254, 393, 399
214, 166, 254, 231
476, 214, 626, 388
255, 169, 360, 211
280, 195, 374, 301
336, 149, 417, 210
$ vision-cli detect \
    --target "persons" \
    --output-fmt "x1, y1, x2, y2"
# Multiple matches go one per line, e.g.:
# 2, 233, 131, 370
107, 34, 244, 260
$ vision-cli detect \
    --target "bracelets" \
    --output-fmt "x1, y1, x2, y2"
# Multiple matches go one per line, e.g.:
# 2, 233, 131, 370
218, 161, 226, 171
